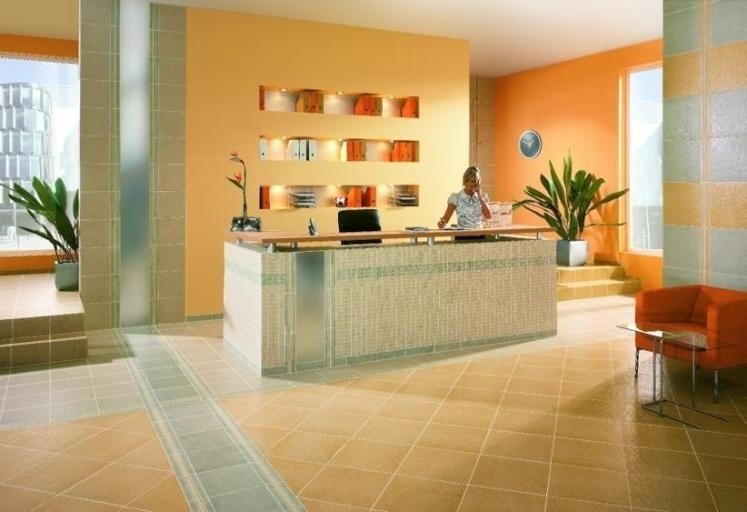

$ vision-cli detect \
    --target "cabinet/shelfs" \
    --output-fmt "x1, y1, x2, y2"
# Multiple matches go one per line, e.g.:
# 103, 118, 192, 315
257, 83, 423, 212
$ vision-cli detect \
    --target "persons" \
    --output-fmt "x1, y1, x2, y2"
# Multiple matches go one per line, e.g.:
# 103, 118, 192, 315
438, 167, 491, 239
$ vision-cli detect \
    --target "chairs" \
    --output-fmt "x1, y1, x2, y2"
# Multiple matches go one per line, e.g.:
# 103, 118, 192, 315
338, 209, 384, 244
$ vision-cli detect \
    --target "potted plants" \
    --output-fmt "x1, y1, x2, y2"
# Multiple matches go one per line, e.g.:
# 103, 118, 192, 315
511, 148, 630, 266
0, 176, 79, 292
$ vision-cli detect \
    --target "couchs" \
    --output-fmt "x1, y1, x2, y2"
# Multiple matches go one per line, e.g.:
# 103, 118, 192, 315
633, 284, 747, 404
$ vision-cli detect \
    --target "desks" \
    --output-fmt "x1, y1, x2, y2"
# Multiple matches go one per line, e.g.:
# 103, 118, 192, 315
616, 315, 745, 431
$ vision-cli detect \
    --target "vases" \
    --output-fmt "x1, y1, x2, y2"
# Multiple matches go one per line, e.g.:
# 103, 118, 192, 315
226, 215, 265, 232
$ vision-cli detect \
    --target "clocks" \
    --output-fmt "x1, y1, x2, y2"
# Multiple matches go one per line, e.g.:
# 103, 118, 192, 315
518, 129, 542, 160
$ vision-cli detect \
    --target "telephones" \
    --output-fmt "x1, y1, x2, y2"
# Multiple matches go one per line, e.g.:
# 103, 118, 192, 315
308, 218, 319, 235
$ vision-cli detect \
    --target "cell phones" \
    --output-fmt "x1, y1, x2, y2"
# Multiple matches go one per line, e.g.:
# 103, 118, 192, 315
478, 179, 481, 183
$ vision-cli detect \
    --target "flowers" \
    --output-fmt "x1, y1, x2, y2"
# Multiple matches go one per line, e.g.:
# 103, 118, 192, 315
227, 150, 250, 213
